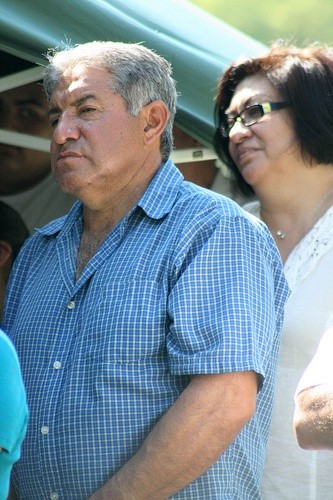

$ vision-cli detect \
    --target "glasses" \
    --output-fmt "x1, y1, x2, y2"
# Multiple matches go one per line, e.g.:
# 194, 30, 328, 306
220, 102, 291, 138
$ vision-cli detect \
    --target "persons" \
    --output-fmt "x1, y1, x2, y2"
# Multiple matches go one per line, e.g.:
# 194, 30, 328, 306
0, 42, 291, 500
293, 327, 333, 451
213, 43, 333, 500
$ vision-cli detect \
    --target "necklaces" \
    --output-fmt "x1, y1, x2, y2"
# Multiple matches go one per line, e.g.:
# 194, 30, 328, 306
261, 189, 333, 240
